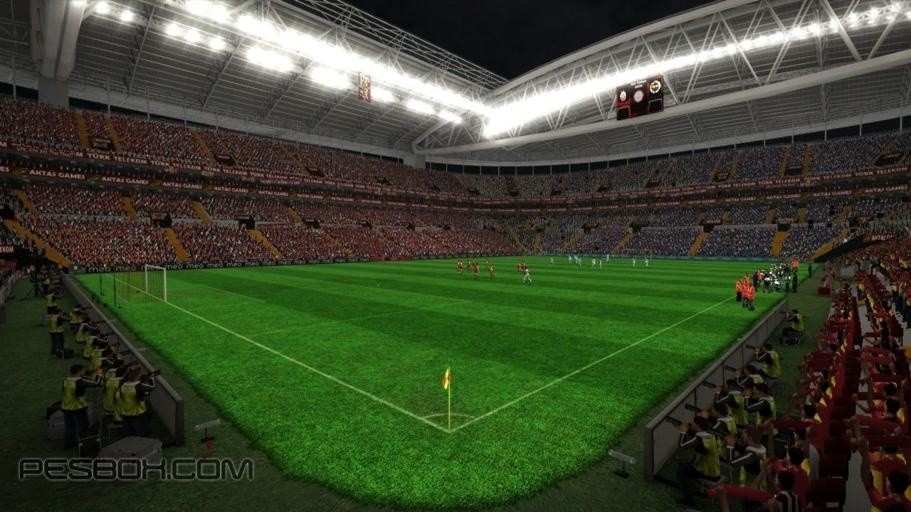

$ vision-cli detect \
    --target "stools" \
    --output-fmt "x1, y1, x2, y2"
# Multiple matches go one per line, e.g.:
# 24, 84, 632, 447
76, 386, 124, 459
697, 473, 728, 510
68, 323, 80, 333
766, 378, 786, 398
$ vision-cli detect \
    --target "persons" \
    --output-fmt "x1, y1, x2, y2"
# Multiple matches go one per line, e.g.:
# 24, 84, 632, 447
0, 99, 911, 512
